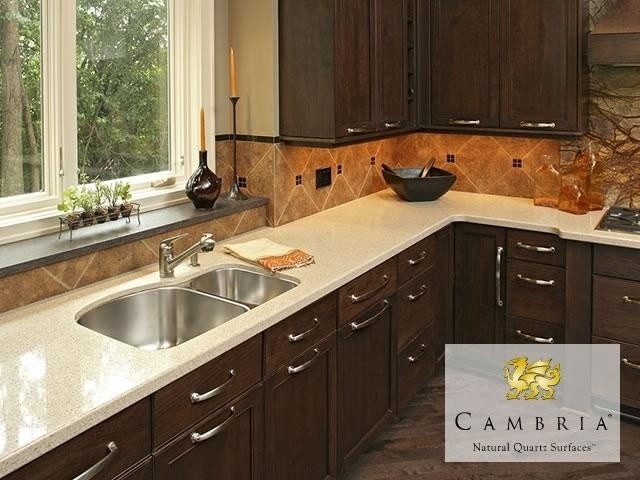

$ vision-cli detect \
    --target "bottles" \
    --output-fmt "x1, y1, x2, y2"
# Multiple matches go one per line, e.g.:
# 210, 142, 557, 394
582, 140, 606, 210
559, 161, 591, 215
533, 154, 562, 208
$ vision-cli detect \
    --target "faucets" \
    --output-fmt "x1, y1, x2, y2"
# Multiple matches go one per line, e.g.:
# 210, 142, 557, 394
157, 229, 216, 277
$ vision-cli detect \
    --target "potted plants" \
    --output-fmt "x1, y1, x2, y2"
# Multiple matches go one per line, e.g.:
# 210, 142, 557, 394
57, 173, 133, 231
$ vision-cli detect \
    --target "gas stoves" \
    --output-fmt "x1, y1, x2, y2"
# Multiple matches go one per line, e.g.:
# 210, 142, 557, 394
594, 206, 640, 235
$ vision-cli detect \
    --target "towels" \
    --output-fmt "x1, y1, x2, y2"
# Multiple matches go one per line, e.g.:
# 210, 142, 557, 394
223, 237, 317, 274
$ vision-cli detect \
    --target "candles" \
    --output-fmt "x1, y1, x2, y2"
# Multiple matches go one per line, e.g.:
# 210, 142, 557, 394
227, 48, 238, 98
199, 104, 205, 151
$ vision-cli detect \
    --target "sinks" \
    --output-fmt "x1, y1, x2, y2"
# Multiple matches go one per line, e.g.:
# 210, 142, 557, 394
75, 282, 250, 351
182, 261, 302, 307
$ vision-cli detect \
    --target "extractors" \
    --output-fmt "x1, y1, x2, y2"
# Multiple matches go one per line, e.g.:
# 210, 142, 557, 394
587, 0, 640, 66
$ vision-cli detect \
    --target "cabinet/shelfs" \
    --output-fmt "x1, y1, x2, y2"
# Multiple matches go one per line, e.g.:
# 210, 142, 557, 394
395, 233, 436, 411
279, 1, 375, 148
436, 220, 456, 376
591, 242, 640, 424
593, 4, 640, 68
506, 227, 593, 413
264, 291, 338, 480
375, 0, 411, 140
151, 332, 266, 479
500, 0, 588, 137
0, 393, 152, 479
424, 0, 500, 134
338, 255, 395, 476
456, 219, 509, 372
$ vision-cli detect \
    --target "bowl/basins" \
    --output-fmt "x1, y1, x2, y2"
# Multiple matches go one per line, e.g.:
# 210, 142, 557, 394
380, 166, 457, 202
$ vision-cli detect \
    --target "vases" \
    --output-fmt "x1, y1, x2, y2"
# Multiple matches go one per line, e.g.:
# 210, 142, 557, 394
185, 150, 222, 211
